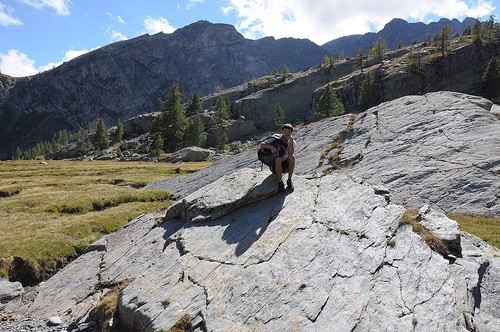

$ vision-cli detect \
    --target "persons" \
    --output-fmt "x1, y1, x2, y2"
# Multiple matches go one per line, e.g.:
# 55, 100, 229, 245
261, 124, 297, 193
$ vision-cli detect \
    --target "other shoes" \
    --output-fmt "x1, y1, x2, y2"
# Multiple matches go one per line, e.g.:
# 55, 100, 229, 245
278, 182, 287, 193
286, 180, 293, 192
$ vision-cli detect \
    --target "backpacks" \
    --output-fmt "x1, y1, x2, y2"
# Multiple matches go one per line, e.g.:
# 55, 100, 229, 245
257, 135, 286, 166
